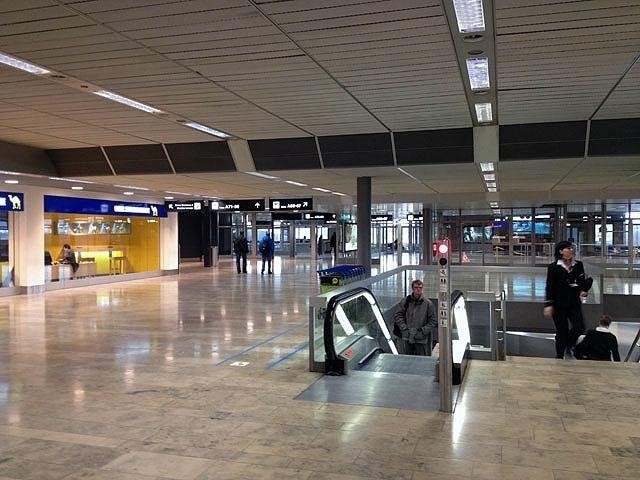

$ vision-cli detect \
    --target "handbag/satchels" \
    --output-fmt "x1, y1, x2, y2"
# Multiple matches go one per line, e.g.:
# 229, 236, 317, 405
391, 295, 412, 337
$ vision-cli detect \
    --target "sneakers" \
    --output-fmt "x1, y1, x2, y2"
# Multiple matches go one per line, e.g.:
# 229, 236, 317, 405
238, 270, 272, 274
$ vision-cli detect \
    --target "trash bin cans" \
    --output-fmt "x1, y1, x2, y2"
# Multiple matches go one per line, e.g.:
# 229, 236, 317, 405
210, 246, 219, 267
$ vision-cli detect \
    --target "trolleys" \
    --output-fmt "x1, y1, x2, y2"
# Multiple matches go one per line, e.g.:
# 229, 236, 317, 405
314, 263, 373, 324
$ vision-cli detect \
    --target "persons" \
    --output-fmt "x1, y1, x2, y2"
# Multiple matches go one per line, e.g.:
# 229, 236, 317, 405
234, 230, 249, 274
390, 239, 397, 252
302, 235, 307, 243
572, 313, 622, 362
58, 243, 79, 280
395, 279, 438, 356
259, 231, 274, 274
317, 233, 324, 259
543, 240, 589, 358
330, 230, 337, 260
44, 249, 52, 283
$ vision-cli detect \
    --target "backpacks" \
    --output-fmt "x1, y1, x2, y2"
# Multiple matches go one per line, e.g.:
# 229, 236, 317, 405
233, 239, 247, 255
262, 239, 271, 254
